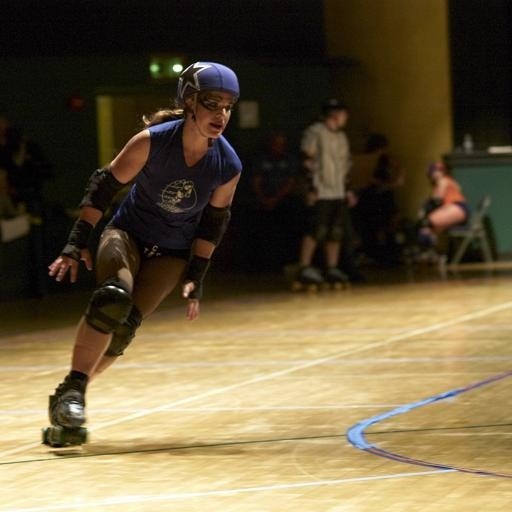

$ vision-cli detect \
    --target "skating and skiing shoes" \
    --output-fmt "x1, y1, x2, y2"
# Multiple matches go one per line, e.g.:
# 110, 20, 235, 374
292, 265, 344, 292
43, 375, 86, 447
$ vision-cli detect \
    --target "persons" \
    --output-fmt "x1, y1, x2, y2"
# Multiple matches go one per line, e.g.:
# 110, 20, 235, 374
37, 58, 243, 428
1, 116, 66, 299
245, 95, 409, 292
406, 159, 471, 264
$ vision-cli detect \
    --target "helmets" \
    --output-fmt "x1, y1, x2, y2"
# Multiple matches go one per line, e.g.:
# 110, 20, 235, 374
177, 62, 240, 102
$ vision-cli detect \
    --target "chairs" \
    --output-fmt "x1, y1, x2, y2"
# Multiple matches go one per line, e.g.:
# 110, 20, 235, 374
446, 195, 497, 265
